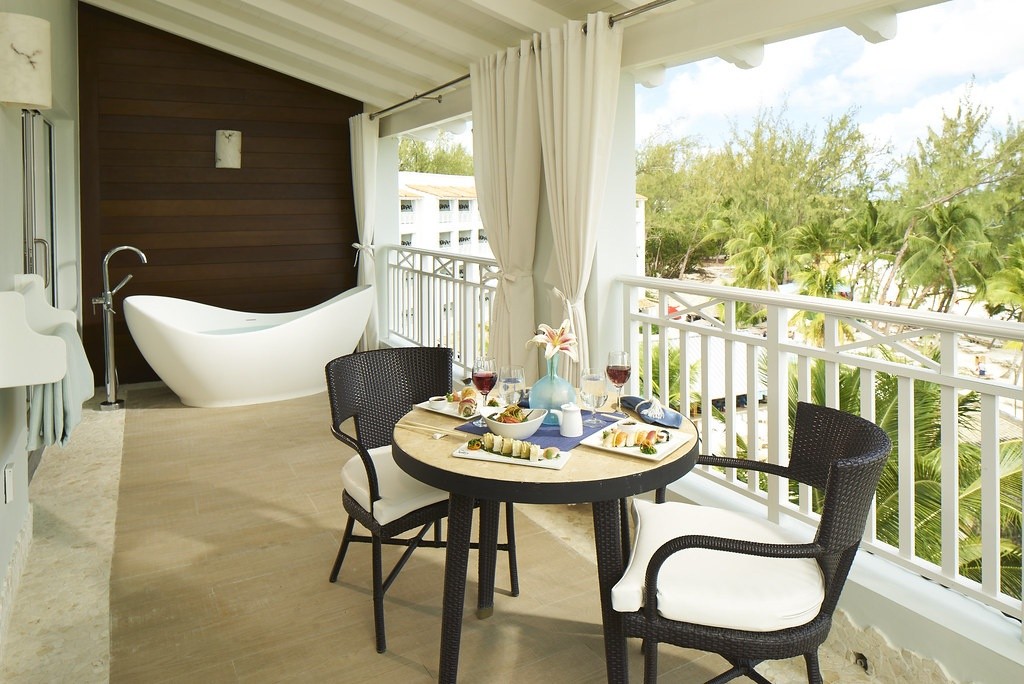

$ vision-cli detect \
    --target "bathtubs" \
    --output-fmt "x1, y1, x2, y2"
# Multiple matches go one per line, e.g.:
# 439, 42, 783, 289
121, 284, 376, 408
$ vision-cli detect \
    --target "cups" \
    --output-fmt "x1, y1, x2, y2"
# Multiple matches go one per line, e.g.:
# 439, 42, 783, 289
497, 365, 525, 406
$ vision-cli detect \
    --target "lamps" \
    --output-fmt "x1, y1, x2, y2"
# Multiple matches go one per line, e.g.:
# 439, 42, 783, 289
215, 130, 242, 169
0, 10, 53, 111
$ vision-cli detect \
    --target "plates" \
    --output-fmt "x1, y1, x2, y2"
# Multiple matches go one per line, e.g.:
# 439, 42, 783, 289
581, 416, 693, 463
453, 440, 571, 470
415, 389, 501, 420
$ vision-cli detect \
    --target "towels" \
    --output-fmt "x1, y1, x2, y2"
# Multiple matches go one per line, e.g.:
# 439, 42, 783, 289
23, 320, 97, 455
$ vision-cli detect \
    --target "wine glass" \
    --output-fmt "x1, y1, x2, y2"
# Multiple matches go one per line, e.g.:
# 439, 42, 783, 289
580, 368, 609, 428
472, 356, 498, 428
605, 351, 632, 418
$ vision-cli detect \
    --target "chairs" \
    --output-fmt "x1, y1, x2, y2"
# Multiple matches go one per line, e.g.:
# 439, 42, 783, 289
610, 401, 893, 684
324, 347, 520, 654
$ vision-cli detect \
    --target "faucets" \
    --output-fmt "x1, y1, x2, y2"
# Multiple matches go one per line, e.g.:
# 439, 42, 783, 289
91, 245, 148, 414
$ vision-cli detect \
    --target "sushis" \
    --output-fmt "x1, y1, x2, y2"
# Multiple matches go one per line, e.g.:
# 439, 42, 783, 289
604, 428, 671, 447
448, 386, 477, 416
471, 433, 540, 461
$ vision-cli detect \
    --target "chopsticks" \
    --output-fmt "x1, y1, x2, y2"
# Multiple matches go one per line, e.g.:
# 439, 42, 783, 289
395, 420, 467, 438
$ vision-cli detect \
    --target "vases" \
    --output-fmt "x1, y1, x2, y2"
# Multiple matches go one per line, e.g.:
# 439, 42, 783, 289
528, 352, 576, 425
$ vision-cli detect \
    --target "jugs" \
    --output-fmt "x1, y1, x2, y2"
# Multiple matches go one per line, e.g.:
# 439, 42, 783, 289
550, 402, 583, 437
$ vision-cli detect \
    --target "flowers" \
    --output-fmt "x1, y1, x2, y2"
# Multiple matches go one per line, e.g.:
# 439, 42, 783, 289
526, 319, 578, 363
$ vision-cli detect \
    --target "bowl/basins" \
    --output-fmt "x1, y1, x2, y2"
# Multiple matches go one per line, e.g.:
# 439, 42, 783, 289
428, 395, 448, 411
616, 420, 638, 431
479, 405, 548, 440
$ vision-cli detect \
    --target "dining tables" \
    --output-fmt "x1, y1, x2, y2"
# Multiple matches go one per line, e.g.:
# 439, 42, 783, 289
392, 386, 700, 684
